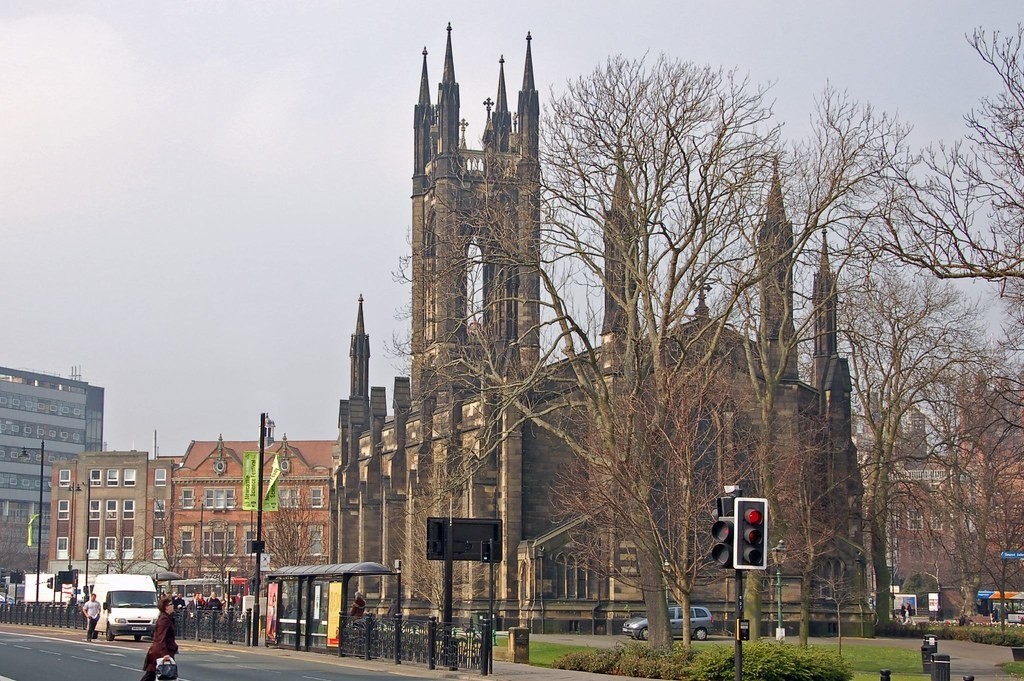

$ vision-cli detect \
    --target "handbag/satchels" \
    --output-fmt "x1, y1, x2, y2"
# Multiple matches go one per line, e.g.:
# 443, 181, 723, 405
156, 657, 179, 681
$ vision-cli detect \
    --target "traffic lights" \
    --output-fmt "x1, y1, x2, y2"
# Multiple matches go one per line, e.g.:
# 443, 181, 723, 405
47, 577, 53, 589
0, 568, 6, 588
711, 516, 734, 570
715, 495, 734, 517
733, 496, 769, 571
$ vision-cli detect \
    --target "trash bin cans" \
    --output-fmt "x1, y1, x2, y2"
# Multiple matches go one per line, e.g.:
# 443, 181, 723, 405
471, 610, 496, 632
921, 645, 937, 674
930, 653, 950, 681
923, 634, 938, 646
958, 617, 965, 626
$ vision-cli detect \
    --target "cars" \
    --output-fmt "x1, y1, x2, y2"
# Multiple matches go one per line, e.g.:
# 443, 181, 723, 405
622, 605, 715, 640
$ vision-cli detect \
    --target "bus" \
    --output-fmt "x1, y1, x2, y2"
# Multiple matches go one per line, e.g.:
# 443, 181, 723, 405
153, 576, 249, 611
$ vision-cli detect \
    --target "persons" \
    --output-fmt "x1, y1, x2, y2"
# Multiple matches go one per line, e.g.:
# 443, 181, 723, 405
900, 602, 916, 625
83, 594, 101, 642
69, 594, 76, 609
139, 599, 178, 681
992, 605, 998, 622
161, 592, 241, 619
936, 606, 944, 621
350, 592, 366, 627
387, 600, 398, 615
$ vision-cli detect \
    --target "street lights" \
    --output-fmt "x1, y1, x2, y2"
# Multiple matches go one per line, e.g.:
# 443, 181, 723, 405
770, 539, 789, 641
66, 481, 74, 571
251, 413, 276, 648
18, 438, 44, 605
75, 477, 93, 603
663, 559, 672, 607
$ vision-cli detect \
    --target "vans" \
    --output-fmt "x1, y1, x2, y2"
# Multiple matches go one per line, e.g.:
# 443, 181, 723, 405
86, 573, 162, 641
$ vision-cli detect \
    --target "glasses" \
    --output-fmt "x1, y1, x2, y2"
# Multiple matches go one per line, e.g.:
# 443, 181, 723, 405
169, 603, 174, 606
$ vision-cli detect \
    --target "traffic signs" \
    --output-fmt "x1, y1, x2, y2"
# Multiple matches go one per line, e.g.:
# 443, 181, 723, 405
1001, 551, 1024, 560
